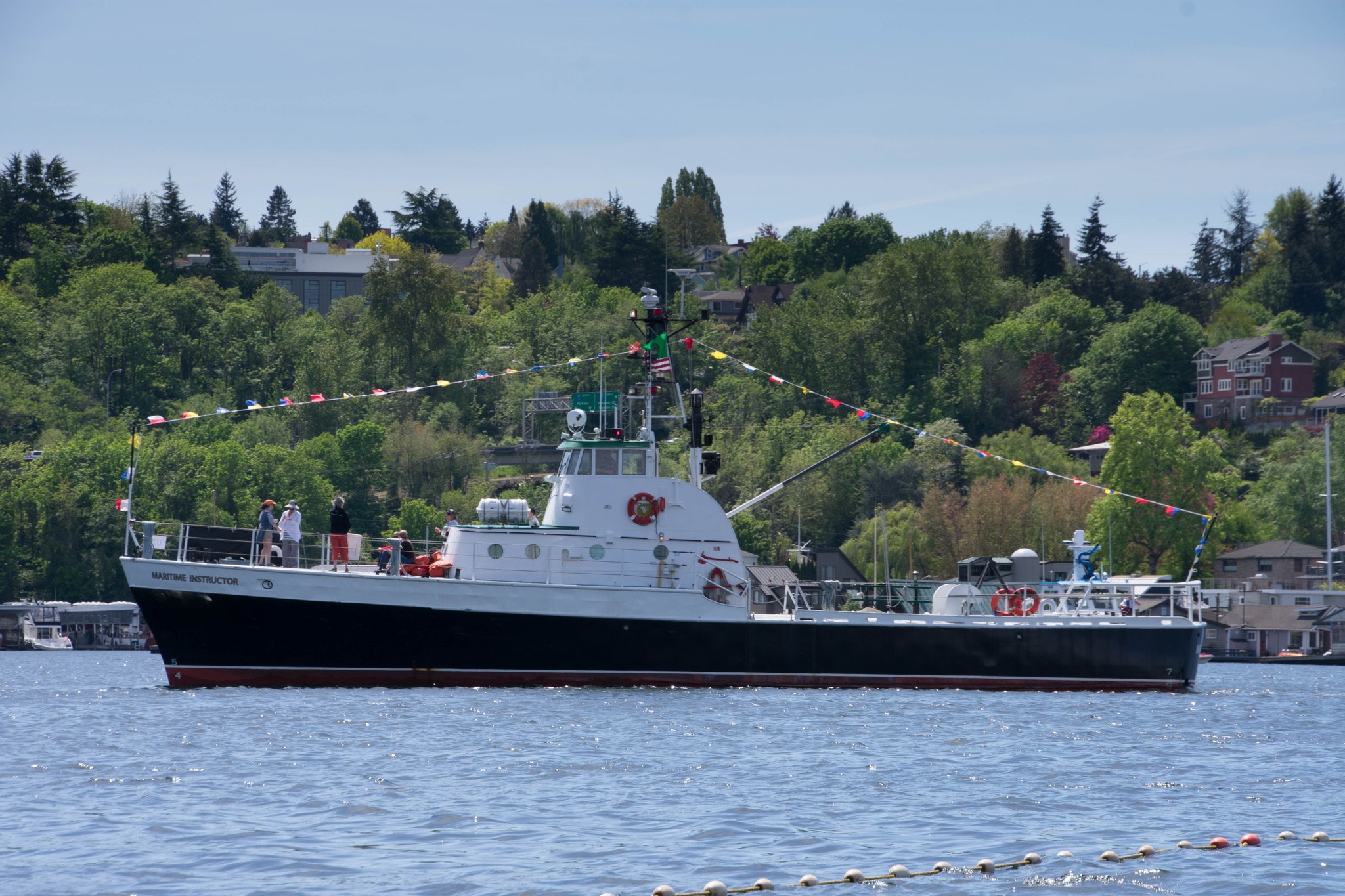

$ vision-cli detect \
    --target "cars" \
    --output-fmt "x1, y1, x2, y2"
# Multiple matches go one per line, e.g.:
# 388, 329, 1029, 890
1322, 649, 1332, 656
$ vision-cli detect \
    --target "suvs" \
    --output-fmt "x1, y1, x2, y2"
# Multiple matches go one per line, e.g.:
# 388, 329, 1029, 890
1276, 648, 1308, 657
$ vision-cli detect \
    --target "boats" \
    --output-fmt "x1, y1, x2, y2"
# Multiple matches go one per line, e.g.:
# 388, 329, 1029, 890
119, 271, 1214, 692
30, 637, 73, 651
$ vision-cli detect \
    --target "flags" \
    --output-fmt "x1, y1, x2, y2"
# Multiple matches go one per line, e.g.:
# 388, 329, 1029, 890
1195, 538, 1205, 553
247, 386, 388, 410
405, 342, 641, 393
684, 338, 899, 426
640, 334, 671, 374
917, 429, 1180, 515
114, 407, 230, 511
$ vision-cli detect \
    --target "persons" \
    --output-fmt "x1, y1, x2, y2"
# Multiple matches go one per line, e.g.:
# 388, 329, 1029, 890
527, 508, 539, 526
374, 530, 415, 573
256, 499, 282, 567
435, 509, 458, 541
1120, 590, 1141, 616
578, 450, 615, 475
277, 500, 302, 568
329, 497, 351, 573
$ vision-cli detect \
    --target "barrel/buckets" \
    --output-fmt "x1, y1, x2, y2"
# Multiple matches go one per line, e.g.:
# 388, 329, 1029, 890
347, 533, 362, 560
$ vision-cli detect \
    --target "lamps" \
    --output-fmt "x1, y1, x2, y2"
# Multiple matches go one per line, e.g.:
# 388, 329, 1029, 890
629, 307, 638, 318
658, 532, 664, 544
560, 432, 570, 440
566, 408, 587, 441
628, 385, 638, 396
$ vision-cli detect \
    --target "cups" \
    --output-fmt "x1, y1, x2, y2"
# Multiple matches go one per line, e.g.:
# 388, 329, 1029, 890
274, 519, 277, 523
285, 515, 289, 518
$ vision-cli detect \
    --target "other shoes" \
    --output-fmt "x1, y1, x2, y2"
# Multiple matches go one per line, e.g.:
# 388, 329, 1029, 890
374, 569, 386, 573
387, 571, 391, 574
344, 568, 349, 572
258, 563, 266, 566
292, 566, 296, 568
266, 563, 277, 567
280, 566, 289, 568
328, 568, 337, 572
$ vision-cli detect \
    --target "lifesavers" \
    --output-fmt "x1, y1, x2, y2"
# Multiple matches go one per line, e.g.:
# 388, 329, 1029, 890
991, 588, 1022, 617
1009, 587, 1040, 617
628, 493, 659, 525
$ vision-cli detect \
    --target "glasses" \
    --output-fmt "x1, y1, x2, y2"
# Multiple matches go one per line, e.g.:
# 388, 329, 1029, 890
393, 536, 398, 538
271, 505, 274, 506
288, 508, 294, 511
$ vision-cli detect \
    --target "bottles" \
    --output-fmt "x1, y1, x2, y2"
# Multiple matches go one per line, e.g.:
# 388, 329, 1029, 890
441, 526, 447, 536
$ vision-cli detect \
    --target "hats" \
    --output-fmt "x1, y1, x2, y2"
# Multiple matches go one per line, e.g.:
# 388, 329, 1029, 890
284, 500, 299, 509
265, 499, 276, 506
